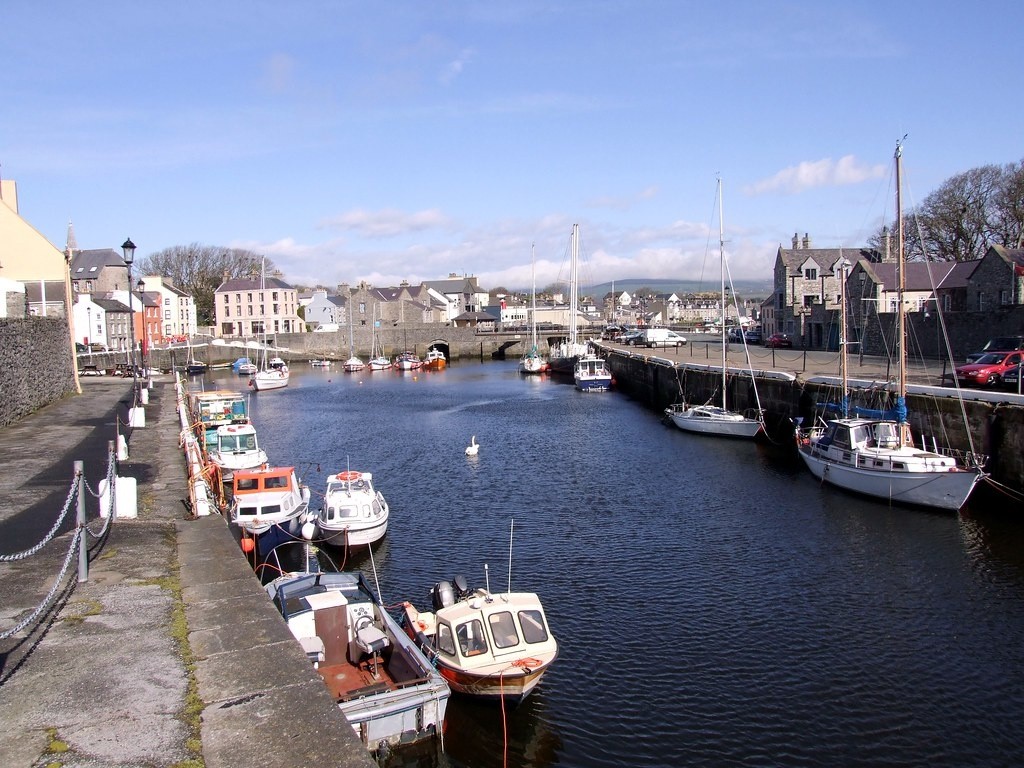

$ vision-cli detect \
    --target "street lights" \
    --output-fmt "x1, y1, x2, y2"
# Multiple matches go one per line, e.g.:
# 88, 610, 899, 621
86, 237, 145, 401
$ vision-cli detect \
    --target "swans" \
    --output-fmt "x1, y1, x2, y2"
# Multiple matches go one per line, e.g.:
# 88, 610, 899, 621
465, 435, 479, 455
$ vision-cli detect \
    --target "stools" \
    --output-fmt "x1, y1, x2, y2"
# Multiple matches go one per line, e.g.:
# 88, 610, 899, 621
354, 625, 391, 681
298, 635, 326, 665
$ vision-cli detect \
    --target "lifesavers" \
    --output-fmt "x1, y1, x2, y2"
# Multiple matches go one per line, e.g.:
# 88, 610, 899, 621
949, 467, 970, 473
595, 370, 604, 376
334, 470, 362, 481
527, 358, 531, 364
226, 424, 254, 432
580, 370, 588, 377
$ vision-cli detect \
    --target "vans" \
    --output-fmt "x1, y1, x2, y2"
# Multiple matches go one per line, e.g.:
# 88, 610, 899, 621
313, 324, 338, 332
644, 329, 688, 348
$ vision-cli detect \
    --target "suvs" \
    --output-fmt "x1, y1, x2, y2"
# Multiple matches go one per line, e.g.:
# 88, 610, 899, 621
966, 335, 1024, 364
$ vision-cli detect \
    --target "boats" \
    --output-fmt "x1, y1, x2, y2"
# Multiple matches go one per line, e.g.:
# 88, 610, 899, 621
189, 377, 389, 553
573, 345, 612, 392
424, 344, 446, 371
78, 331, 250, 377
260, 517, 452, 755
208, 362, 232, 368
401, 517, 561, 711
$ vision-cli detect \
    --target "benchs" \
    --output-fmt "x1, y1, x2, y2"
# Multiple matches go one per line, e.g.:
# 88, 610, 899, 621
120, 370, 127, 378
137, 371, 143, 377
95, 367, 102, 375
78, 368, 84, 376
110, 370, 115, 376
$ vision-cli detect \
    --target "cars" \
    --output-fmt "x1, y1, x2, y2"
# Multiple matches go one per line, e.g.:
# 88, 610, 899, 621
954, 350, 1024, 393
729, 328, 792, 349
76, 342, 106, 353
605, 329, 645, 344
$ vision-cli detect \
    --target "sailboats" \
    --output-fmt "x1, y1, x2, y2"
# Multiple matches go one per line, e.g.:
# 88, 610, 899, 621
664, 176, 765, 438
239, 256, 289, 391
789, 133, 988, 512
518, 223, 595, 374
309, 291, 423, 370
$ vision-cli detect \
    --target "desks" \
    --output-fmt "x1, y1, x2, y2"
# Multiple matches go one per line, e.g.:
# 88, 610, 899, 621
126, 364, 138, 377
104, 368, 114, 375
115, 363, 128, 376
83, 364, 98, 376
